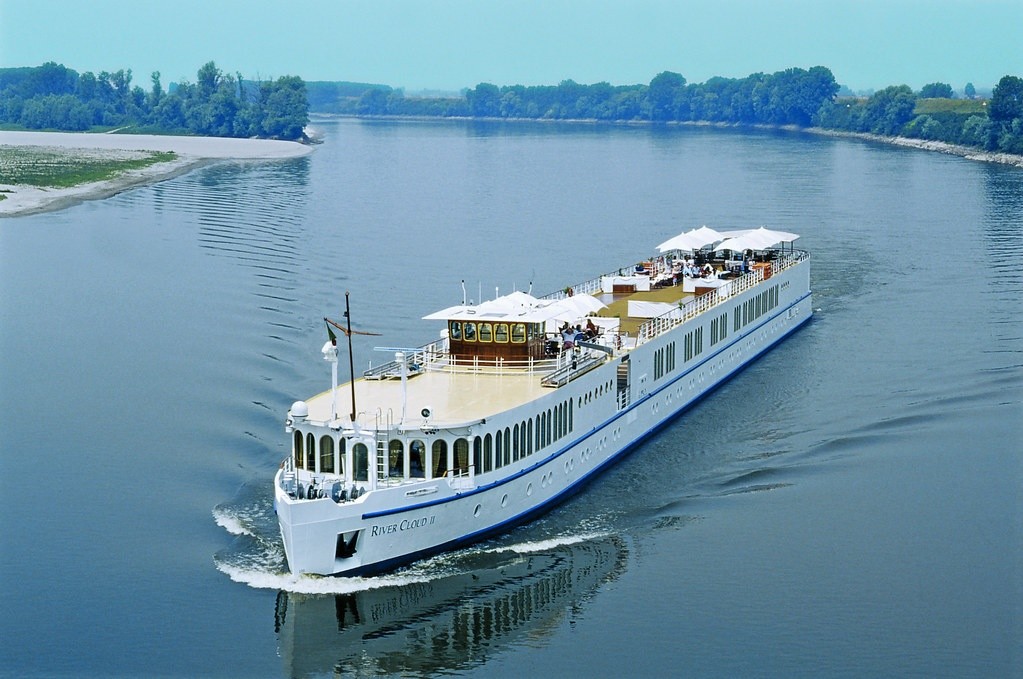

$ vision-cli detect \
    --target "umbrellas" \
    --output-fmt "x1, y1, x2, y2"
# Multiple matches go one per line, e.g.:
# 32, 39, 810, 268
474, 291, 610, 324
655, 224, 800, 253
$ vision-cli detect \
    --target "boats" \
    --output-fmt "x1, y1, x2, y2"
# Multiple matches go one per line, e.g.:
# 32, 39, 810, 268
275, 225, 814, 580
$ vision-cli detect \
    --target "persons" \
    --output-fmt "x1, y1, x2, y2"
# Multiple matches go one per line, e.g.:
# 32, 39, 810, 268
672, 274, 679, 288
672, 263, 704, 278
559, 319, 595, 353
613, 334, 622, 350
